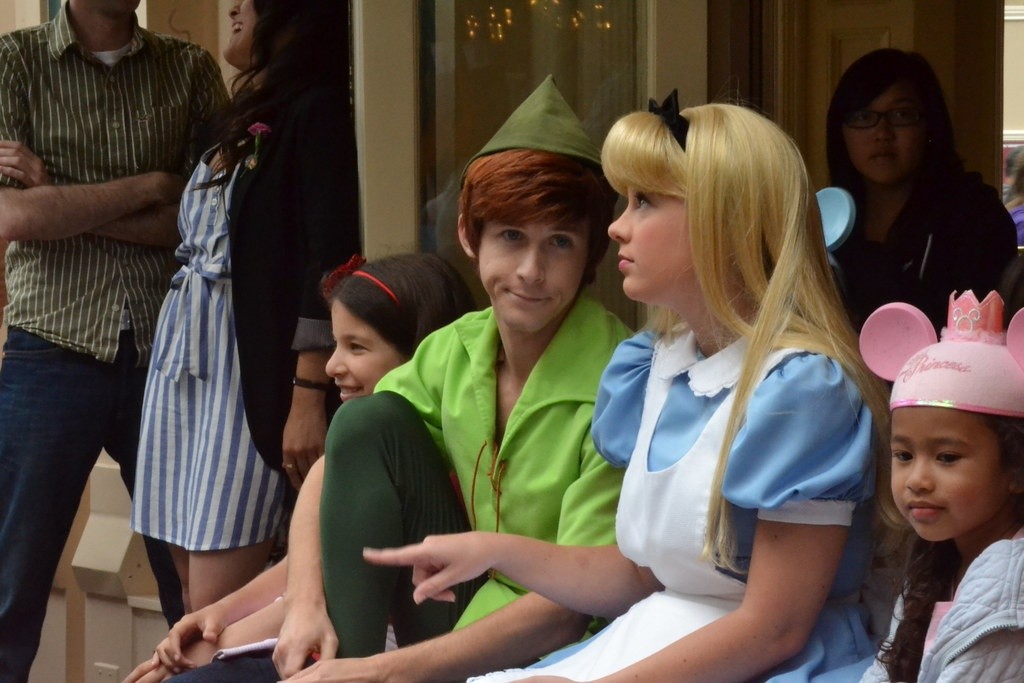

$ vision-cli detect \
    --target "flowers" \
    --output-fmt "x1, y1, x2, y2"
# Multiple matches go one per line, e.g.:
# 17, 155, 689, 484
238, 121, 270, 177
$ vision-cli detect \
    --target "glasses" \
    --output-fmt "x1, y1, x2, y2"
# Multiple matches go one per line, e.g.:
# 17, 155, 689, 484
843, 106, 920, 129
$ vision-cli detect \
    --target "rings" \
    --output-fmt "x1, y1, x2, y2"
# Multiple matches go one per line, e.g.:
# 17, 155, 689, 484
281, 463, 294, 468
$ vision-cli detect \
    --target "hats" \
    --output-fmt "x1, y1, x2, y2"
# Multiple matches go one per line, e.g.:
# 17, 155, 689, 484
857, 287, 1024, 418
458, 75, 602, 189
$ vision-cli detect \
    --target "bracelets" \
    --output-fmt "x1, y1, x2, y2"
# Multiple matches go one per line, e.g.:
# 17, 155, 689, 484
292, 377, 329, 391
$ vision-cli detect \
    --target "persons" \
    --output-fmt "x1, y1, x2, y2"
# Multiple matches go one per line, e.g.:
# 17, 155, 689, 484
1001, 160, 1024, 253
822, 47, 1018, 338
0, 0, 230, 683
849, 291, 1024, 683
270, 79, 635, 683
130, 1, 359, 612
359, 87, 894, 683
123, 251, 476, 683
